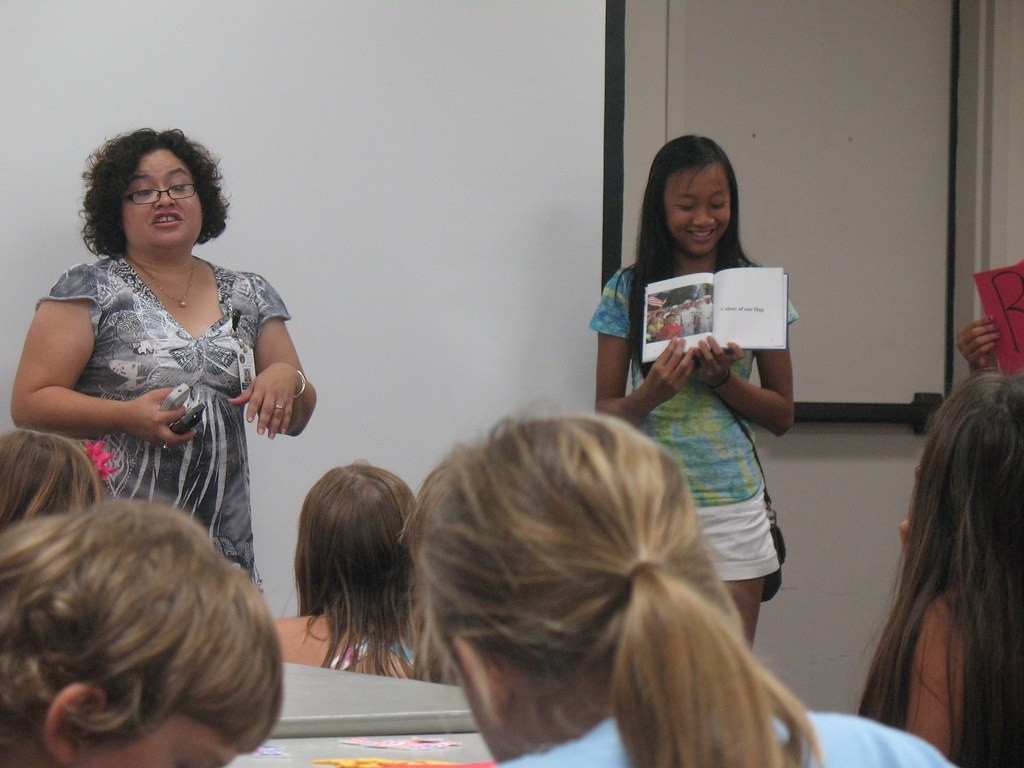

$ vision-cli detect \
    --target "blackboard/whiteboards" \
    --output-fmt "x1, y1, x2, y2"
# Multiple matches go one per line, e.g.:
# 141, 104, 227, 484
0, 1, 627, 617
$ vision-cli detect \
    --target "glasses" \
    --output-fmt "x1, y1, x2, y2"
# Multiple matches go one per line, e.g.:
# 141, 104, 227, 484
122, 183, 199, 205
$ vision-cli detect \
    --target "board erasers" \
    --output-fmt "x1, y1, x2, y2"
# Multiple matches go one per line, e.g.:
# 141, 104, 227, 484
159, 383, 189, 412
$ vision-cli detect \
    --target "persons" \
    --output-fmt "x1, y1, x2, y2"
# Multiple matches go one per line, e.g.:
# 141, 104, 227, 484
857, 372, 1024, 767
275, 464, 418, 679
0, 432, 283, 767
418, 416, 956, 767
587, 136, 799, 649
956, 315, 1000, 372
245, 370, 251, 382
10, 129, 317, 588
647, 295, 713, 343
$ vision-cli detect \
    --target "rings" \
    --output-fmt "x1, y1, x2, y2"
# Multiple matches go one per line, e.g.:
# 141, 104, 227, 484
275, 405, 284, 409
163, 442, 167, 449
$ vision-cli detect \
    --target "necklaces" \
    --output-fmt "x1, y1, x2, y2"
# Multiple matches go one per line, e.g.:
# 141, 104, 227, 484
136, 264, 193, 307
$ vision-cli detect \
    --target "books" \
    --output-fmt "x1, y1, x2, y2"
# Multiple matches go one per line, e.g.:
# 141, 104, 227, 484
641, 268, 788, 364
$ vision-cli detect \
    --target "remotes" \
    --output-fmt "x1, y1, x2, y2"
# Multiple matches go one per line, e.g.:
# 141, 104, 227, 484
161, 383, 190, 411
169, 404, 205, 435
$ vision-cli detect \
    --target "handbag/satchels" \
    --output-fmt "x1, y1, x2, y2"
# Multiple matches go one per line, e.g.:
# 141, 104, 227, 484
761, 524, 785, 602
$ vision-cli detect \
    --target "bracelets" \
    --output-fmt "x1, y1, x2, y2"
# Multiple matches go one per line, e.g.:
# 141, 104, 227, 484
294, 370, 306, 397
706, 368, 730, 389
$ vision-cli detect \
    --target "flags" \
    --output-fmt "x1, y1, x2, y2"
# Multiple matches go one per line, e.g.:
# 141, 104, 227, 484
648, 294, 662, 307
